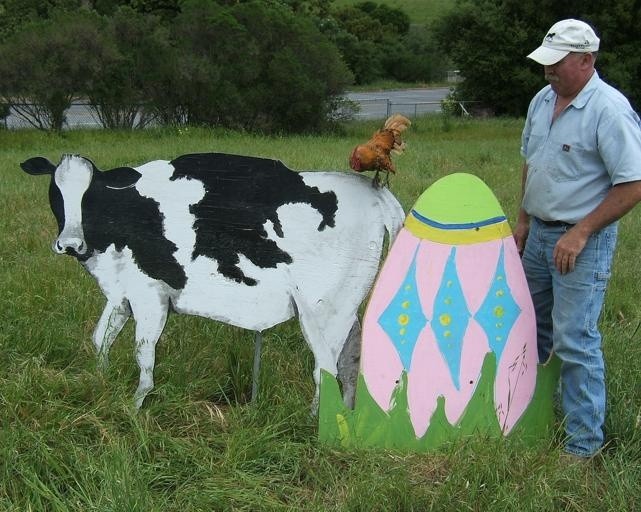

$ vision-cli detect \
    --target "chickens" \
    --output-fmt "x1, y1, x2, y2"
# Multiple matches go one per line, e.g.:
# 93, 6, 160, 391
349, 113, 412, 190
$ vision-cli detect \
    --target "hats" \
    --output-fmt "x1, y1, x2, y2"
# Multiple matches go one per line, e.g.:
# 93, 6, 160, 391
527, 18, 601, 66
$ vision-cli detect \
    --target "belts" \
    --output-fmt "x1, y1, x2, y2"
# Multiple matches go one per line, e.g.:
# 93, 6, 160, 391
536, 217, 572, 227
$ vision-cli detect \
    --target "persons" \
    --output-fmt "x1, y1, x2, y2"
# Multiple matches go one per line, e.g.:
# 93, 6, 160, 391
511, 18, 641, 468
